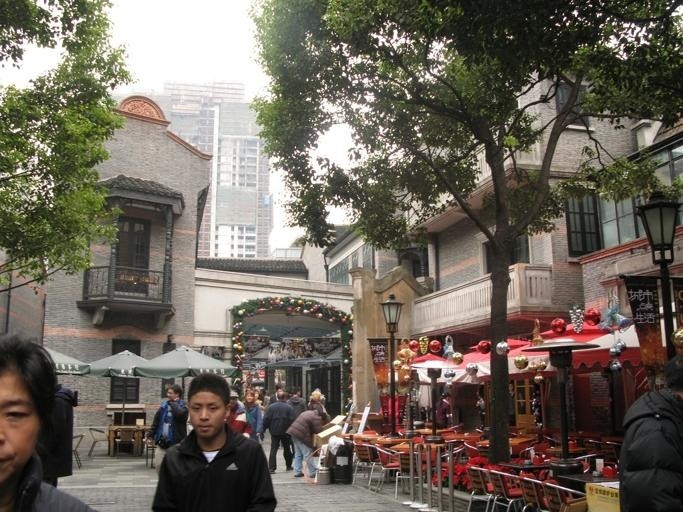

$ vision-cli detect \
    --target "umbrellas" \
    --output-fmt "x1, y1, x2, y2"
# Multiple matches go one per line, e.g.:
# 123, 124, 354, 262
135, 345, 240, 404
85, 347, 149, 426
42, 347, 91, 378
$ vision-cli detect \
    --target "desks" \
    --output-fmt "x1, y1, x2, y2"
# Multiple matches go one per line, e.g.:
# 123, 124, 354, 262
109, 425, 152, 458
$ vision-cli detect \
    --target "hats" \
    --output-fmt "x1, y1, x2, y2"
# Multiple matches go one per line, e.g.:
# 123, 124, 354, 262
230, 390, 238, 398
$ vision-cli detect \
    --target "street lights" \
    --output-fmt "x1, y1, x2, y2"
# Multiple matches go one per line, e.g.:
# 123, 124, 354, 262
379, 294, 407, 436
632, 185, 683, 362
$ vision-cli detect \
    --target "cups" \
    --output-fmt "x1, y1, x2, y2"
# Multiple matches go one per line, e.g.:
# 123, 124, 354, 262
524, 459, 531, 464
592, 471, 601, 477
596, 458, 604, 474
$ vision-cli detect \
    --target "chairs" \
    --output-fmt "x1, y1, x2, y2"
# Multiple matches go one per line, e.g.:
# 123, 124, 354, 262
352, 430, 622, 512
72, 434, 83, 468
144, 428, 157, 468
88, 428, 110, 457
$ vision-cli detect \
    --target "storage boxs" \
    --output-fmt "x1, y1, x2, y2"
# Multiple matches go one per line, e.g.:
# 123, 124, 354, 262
316, 415, 346, 449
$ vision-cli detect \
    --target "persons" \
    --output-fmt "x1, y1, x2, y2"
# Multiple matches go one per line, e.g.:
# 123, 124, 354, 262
225, 382, 331, 484
143, 384, 191, 481
151, 375, 278, 511
37, 382, 79, 488
434, 390, 452, 429
1, 330, 97, 511
618, 354, 683, 512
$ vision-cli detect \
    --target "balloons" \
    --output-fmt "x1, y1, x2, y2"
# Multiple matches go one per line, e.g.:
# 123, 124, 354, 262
670, 326, 683, 351
391, 307, 628, 385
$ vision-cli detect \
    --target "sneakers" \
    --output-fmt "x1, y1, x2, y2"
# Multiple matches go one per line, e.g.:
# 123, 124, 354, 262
294, 474, 304, 477
269, 468, 274, 473
286, 466, 293, 470
